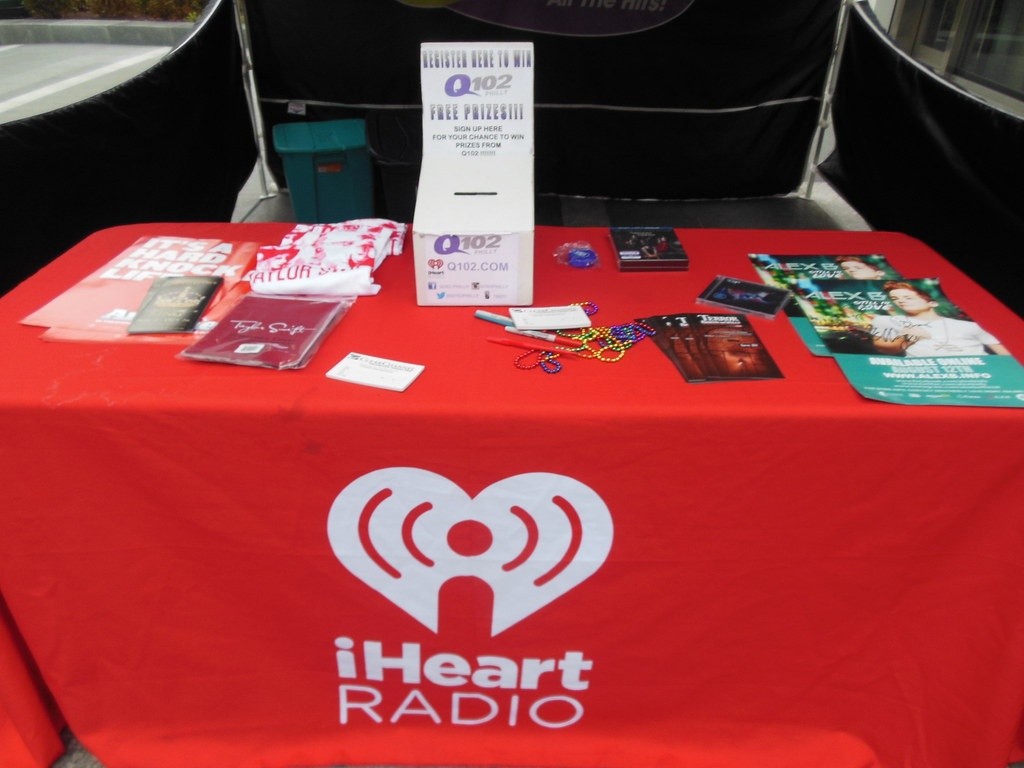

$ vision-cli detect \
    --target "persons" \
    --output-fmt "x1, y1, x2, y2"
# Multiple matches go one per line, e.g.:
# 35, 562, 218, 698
872, 282, 1011, 357
836, 253, 884, 280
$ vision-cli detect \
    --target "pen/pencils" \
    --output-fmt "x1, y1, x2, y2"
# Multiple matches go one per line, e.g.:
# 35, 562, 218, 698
504, 325, 583, 348
487, 337, 578, 355
473, 308, 516, 326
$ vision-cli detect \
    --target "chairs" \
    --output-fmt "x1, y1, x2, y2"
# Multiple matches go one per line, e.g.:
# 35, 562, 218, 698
0, 0, 258, 297
229, 1, 843, 229
807, 0, 1024, 314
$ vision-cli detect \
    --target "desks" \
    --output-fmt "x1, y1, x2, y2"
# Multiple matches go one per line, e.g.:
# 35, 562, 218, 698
0, 231, 1024, 768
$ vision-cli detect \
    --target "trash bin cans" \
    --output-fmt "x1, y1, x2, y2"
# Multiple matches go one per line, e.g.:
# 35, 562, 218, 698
271, 119, 375, 224
